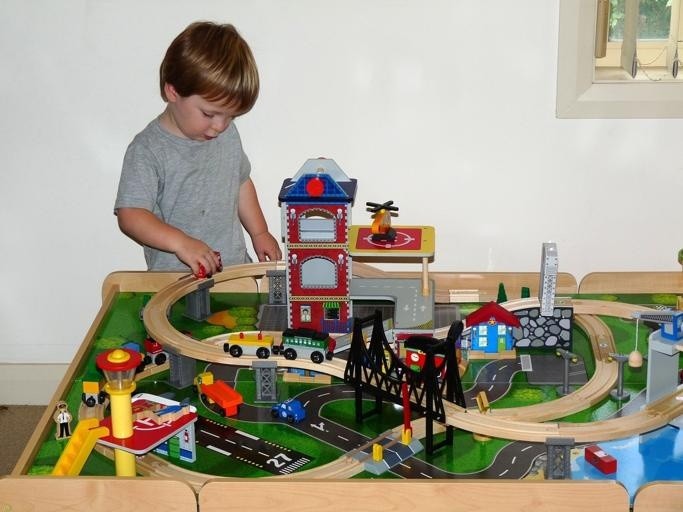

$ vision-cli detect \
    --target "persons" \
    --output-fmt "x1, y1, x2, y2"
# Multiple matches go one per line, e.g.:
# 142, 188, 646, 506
113, 21, 282, 278
